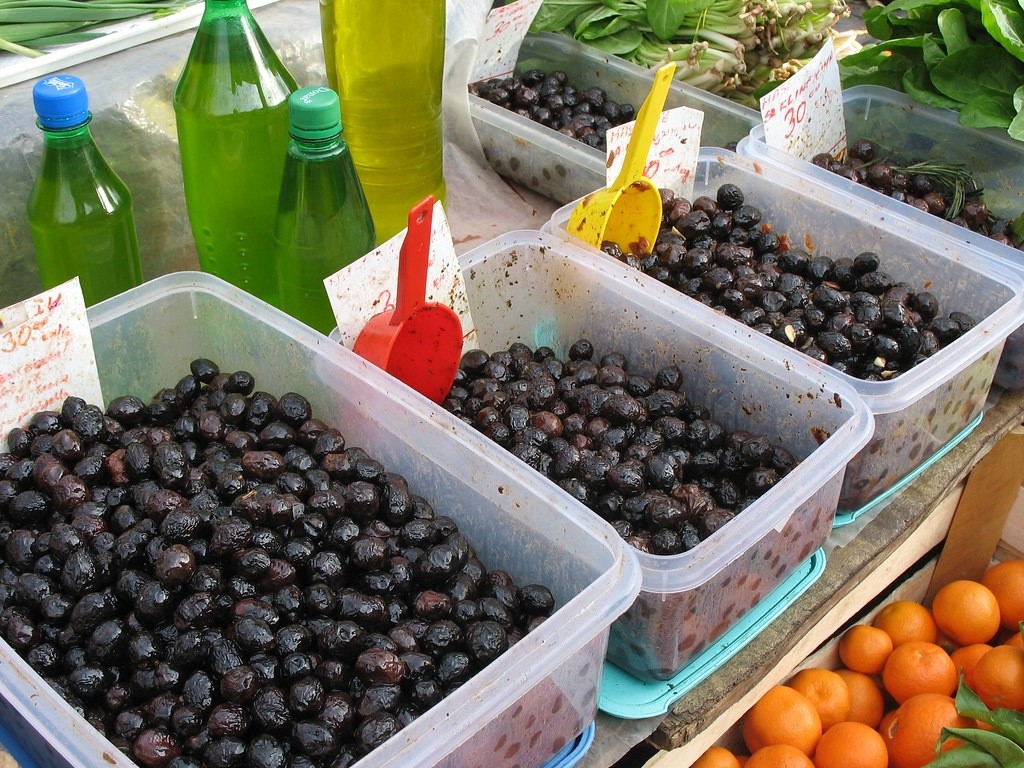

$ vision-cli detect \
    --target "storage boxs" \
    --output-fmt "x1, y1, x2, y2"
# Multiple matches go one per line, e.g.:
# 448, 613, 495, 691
0, 26, 1024, 768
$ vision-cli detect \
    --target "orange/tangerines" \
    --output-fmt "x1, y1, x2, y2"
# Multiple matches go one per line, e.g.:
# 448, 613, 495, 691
686, 558, 1024, 768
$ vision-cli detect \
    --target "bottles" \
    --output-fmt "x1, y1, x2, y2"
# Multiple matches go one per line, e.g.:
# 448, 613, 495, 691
273, 85, 377, 336
171, 1, 298, 309
318, 1, 449, 241
29, 74, 146, 309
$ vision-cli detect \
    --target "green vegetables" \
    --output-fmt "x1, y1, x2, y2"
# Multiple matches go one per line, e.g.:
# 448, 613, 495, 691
829, 0, 1024, 145
495, 0, 851, 111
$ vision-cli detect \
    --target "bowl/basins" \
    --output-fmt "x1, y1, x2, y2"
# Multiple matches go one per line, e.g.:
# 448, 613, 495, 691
0, 32, 1024, 767
